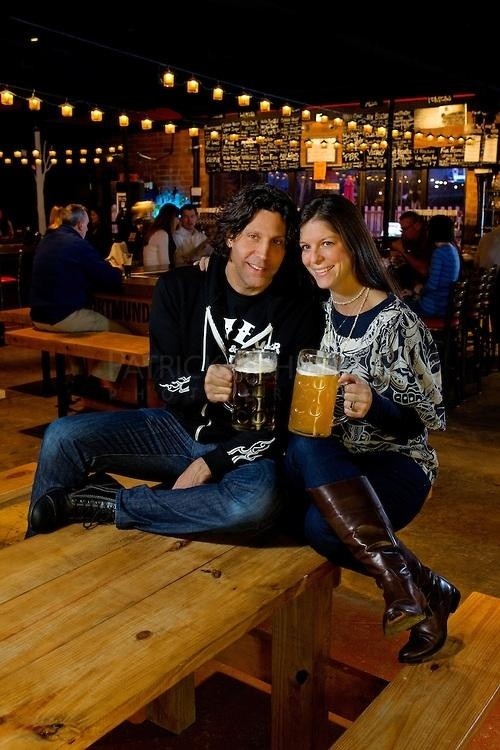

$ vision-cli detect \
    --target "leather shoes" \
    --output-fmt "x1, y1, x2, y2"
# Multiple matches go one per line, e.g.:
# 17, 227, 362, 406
28, 472, 126, 534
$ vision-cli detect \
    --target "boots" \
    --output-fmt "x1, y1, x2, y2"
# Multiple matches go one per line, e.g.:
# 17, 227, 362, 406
304, 474, 460, 663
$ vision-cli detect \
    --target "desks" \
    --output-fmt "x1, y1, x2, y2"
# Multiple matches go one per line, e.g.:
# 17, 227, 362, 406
0, 522, 342, 749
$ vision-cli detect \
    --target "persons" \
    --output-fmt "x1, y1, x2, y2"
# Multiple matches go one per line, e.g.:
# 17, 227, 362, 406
387, 211, 500, 318
0, 210, 14, 241
86, 203, 215, 270
29, 203, 131, 400
24, 183, 325, 540
286, 193, 461, 663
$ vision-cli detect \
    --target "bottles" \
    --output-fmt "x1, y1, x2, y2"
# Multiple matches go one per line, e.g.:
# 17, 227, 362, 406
365, 206, 463, 237
173, 185, 178, 198
494, 172, 500, 209
268, 171, 289, 189
197, 208, 220, 224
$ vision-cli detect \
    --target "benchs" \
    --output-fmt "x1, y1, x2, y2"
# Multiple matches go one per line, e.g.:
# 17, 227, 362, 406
328, 589, 499, 750
0, 305, 32, 327
1, 319, 150, 416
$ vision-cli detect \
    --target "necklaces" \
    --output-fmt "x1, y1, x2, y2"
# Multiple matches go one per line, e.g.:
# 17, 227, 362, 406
328, 286, 369, 357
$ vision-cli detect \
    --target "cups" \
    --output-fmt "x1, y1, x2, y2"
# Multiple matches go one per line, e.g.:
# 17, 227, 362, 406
288, 350, 351, 439
122, 253, 133, 278
223, 348, 278, 433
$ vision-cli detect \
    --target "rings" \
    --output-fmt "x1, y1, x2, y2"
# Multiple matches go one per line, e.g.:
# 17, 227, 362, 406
350, 402, 356, 410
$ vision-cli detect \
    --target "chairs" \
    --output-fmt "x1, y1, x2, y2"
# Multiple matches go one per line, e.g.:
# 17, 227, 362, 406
459, 264, 498, 365
418, 276, 474, 383
